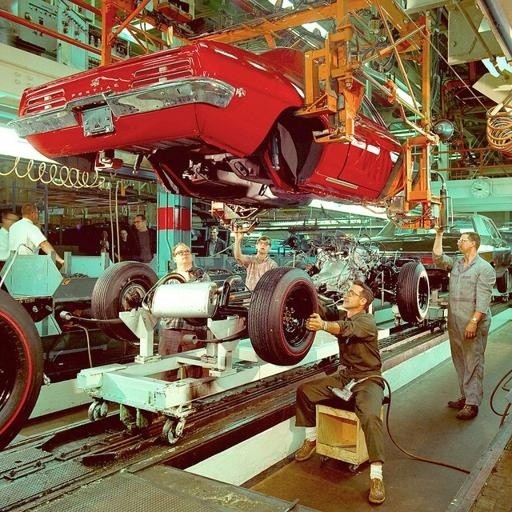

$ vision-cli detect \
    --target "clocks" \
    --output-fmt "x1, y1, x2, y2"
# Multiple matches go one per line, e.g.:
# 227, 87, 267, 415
470, 179, 491, 199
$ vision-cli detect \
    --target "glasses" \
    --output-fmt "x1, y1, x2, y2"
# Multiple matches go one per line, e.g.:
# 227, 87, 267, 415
458, 239, 469, 243
345, 290, 360, 297
2, 213, 18, 222
35, 210, 39, 213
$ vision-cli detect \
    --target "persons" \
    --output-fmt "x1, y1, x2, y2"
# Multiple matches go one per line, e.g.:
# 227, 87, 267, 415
203, 225, 228, 258
431, 226, 496, 423
8, 202, 65, 265
95, 229, 112, 260
157, 241, 212, 382
232, 226, 280, 306
125, 214, 156, 264
0, 209, 20, 292
294, 280, 389, 508
113, 228, 132, 262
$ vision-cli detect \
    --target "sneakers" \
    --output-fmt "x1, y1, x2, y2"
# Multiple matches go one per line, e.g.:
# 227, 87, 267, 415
456, 404, 478, 419
448, 398, 466, 409
369, 477, 386, 504
295, 438, 316, 461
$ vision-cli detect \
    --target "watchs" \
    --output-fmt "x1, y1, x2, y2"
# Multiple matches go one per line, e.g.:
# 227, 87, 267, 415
322, 320, 328, 331
470, 316, 480, 323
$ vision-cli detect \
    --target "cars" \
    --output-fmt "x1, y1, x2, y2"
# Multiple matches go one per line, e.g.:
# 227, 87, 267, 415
17, 40, 421, 210
359, 210, 512, 294
